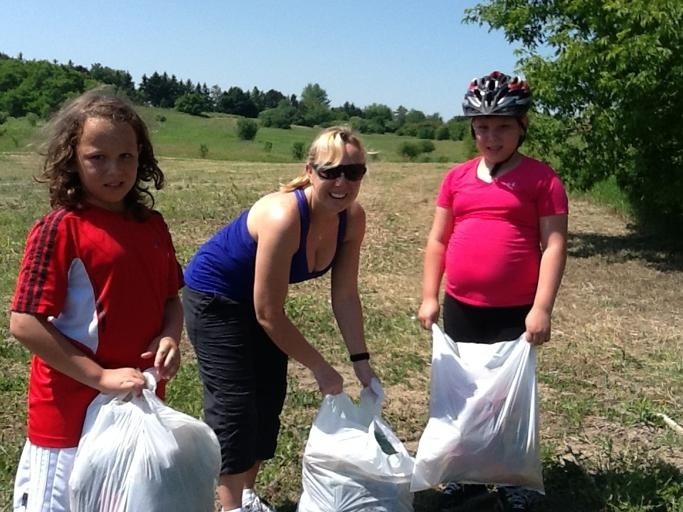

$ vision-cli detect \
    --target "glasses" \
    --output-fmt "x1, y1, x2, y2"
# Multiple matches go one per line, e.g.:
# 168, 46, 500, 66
311, 163, 368, 180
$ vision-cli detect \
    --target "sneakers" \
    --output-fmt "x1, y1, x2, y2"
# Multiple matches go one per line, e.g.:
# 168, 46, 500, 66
243, 496, 275, 512
439, 482, 527, 512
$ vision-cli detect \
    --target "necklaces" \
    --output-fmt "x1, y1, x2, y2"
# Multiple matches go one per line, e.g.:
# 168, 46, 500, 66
310, 223, 329, 242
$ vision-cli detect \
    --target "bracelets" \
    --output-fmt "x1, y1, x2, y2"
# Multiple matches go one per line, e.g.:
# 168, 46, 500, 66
349, 352, 369, 362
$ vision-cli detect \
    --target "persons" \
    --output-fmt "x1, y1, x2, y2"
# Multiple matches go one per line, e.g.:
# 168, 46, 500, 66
181, 124, 382, 512
417, 72, 569, 512
10, 86, 183, 512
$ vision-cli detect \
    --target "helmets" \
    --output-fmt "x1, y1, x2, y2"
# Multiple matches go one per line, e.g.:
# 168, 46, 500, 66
461, 71, 532, 115
